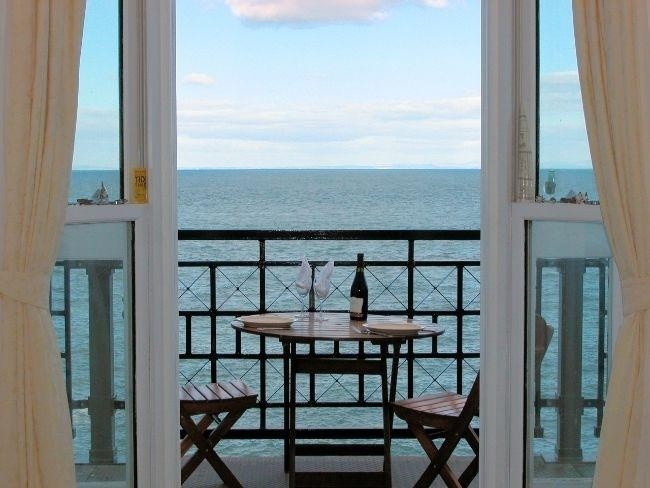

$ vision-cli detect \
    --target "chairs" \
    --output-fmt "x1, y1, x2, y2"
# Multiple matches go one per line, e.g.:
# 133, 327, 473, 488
180, 380, 258, 488
390, 314, 554, 488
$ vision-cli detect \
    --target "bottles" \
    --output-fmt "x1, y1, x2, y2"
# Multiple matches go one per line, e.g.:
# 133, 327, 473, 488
544, 168, 557, 196
348, 253, 369, 322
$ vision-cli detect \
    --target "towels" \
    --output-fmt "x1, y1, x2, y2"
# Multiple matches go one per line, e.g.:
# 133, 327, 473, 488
296, 257, 334, 298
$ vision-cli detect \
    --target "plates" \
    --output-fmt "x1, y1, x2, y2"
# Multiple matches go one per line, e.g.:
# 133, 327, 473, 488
361, 322, 426, 337
233, 313, 299, 328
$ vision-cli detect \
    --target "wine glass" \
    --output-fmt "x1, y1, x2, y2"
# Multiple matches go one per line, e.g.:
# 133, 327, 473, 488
313, 278, 331, 321
295, 280, 312, 319
